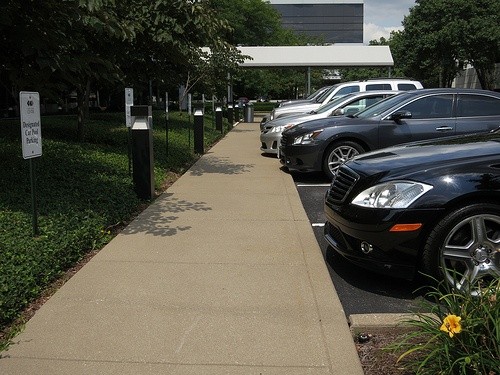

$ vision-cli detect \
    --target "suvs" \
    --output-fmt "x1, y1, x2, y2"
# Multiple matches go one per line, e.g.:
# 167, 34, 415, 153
259, 76, 423, 157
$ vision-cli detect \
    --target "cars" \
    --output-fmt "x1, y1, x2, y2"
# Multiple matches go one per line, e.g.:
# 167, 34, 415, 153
321, 125, 500, 301
282, 87, 499, 181
